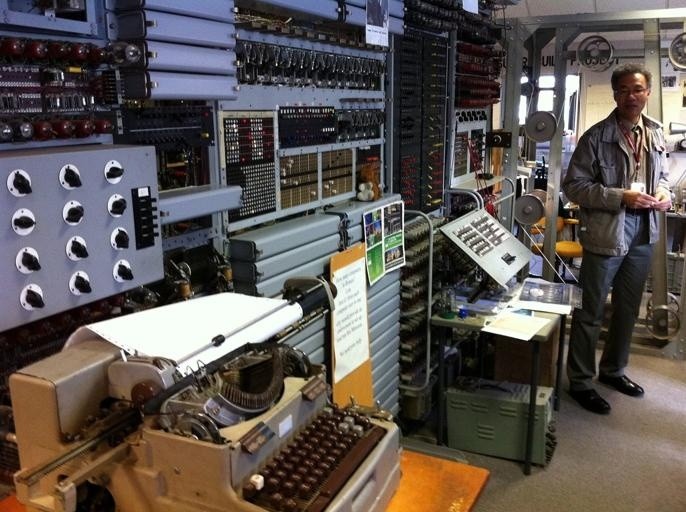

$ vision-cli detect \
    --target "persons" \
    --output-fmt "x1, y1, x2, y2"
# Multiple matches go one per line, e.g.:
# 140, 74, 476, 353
562, 63, 675, 414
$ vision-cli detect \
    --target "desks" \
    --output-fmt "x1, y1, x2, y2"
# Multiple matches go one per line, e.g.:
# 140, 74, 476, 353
431, 281, 567, 476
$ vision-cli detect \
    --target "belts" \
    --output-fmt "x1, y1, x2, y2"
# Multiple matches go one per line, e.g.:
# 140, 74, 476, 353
627, 207, 645, 216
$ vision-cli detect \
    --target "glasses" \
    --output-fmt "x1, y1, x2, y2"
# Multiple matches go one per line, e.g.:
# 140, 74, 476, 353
614, 85, 649, 97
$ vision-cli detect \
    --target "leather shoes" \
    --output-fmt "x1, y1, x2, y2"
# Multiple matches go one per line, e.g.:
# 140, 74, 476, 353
599, 371, 644, 396
568, 385, 610, 414
